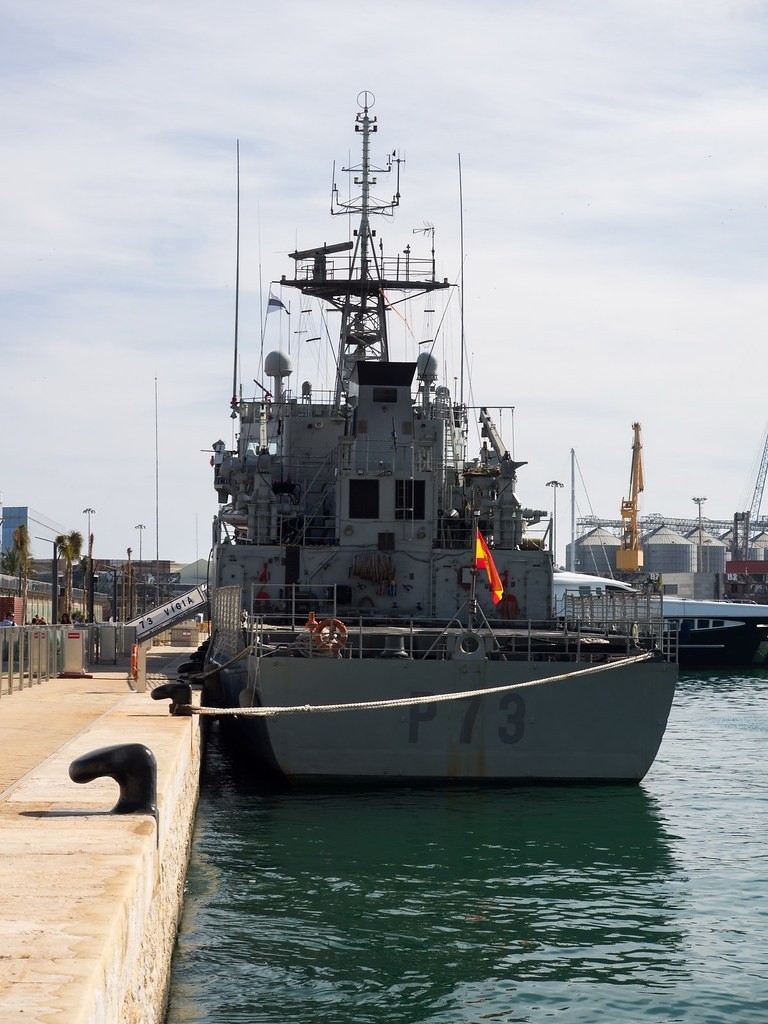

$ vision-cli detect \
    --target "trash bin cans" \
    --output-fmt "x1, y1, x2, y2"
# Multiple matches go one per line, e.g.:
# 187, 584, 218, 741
99, 626, 117, 665
64, 630, 89, 675
123, 626, 136, 657
28, 629, 54, 675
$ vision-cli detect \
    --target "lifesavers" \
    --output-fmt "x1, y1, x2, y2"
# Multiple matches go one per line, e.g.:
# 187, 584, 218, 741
130, 644, 139, 677
312, 617, 348, 651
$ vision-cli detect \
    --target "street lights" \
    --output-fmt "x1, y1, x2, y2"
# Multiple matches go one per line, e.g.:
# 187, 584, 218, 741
545, 480, 566, 568
83, 508, 96, 537
134, 524, 147, 572
692, 495, 707, 572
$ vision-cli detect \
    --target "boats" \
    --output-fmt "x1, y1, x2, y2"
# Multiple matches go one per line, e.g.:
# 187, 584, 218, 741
544, 422, 768, 667
151, 89, 684, 782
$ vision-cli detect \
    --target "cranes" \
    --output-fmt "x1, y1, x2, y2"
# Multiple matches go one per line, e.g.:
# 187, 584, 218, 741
616, 421, 647, 571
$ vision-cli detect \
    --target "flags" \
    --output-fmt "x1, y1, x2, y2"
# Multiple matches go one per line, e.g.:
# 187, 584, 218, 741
476, 528, 504, 607
267, 290, 291, 315
